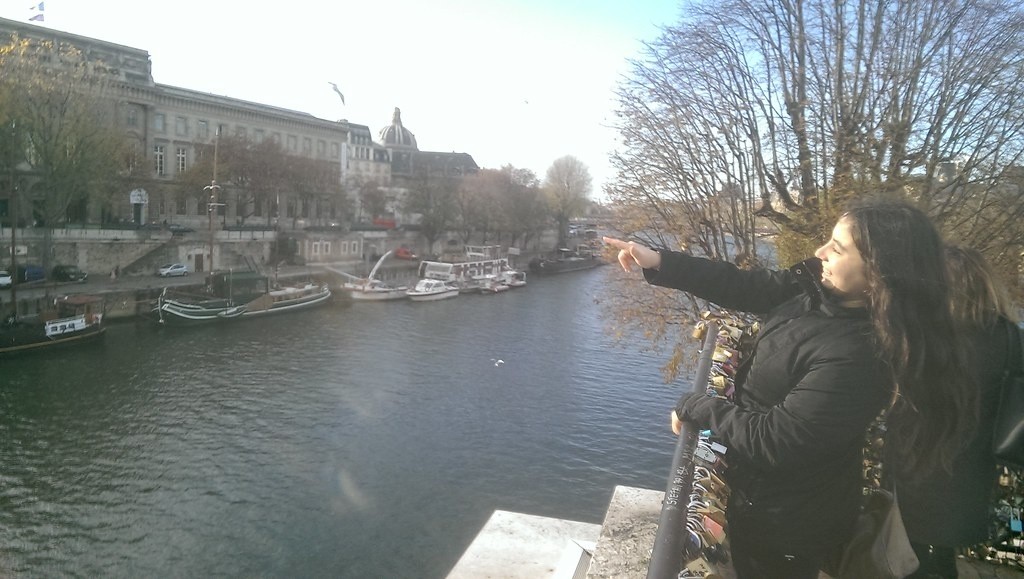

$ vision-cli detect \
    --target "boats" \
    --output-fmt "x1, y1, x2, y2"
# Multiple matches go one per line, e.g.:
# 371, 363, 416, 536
0, 294, 106, 352
530, 249, 595, 275
340, 251, 408, 300
418, 258, 527, 292
406, 279, 460, 301
151, 267, 331, 326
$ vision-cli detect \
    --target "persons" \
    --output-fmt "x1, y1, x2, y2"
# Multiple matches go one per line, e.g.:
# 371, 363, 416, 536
602, 204, 958, 579
883, 243, 1024, 579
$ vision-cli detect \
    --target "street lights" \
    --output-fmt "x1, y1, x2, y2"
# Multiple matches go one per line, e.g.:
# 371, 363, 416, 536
201, 126, 227, 284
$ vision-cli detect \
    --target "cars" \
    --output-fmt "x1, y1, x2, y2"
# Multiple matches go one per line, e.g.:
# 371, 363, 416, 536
393, 247, 418, 259
0, 270, 13, 289
156, 263, 188, 277
55, 265, 88, 282
18, 265, 43, 282
169, 224, 194, 232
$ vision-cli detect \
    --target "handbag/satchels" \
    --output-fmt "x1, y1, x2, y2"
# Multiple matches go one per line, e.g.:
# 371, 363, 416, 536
820, 485, 920, 579
991, 315, 1024, 471
